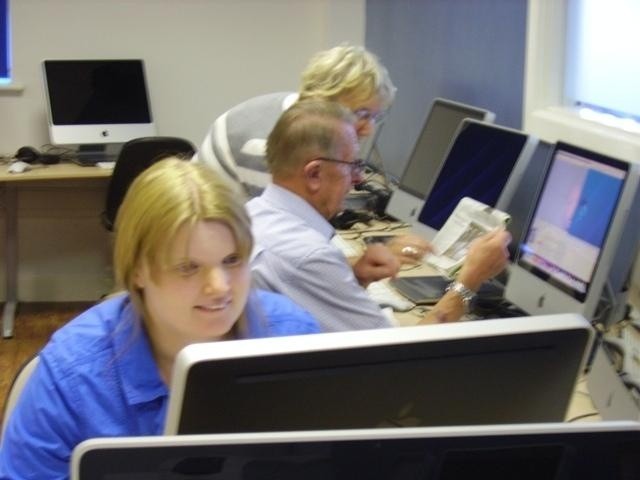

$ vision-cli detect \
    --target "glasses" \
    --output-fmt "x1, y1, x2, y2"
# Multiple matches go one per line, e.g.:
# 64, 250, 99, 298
306, 157, 364, 174
356, 108, 386, 124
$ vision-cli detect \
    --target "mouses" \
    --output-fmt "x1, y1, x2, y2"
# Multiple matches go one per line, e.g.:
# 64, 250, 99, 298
9, 161, 32, 173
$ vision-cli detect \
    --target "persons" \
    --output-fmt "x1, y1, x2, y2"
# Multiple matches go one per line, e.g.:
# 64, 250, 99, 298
0, 155, 326, 480
243, 99, 513, 335
192, 44, 397, 202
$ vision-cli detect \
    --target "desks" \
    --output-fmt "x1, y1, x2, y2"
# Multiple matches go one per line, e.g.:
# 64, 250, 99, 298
333, 170, 639, 422
1, 159, 114, 340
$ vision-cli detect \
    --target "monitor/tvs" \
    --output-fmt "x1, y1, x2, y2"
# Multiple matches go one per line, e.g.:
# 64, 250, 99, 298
411, 117, 540, 242
41, 58, 157, 166
356, 87, 398, 169
163, 313, 596, 435
503, 141, 640, 323
384, 98, 497, 223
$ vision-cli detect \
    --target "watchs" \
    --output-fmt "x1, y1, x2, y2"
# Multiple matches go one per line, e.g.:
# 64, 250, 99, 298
446, 280, 478, 312
